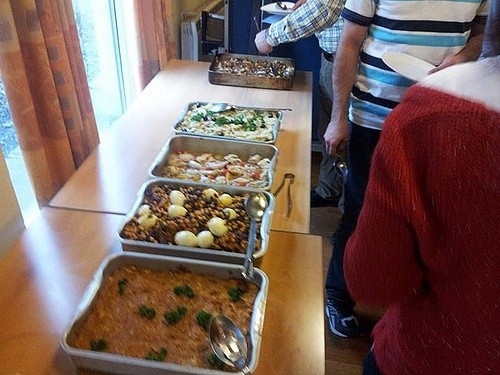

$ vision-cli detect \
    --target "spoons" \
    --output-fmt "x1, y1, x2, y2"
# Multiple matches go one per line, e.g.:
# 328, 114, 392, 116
277, 2, 292, 10
333, 152, 349, 178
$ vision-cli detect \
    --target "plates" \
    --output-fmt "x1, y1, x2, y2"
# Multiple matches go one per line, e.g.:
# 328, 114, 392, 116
260, 2, 296, 15
382, 51, 437, 82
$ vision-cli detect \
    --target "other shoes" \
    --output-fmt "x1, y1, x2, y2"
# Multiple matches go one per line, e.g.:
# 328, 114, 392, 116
311, 191, 340, 207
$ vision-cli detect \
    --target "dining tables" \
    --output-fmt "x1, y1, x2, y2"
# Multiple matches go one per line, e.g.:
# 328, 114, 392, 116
45, 58, 313, 235
0, 207, 325, 375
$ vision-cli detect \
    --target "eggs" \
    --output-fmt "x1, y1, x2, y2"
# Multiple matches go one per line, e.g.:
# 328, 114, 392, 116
167, 205, 188, 219
169, 190, 185, 206
208, 217, 228, 237
202, 188, 237, 220
138, 204, 157, 227
174, 230, 198, 247
194, 230, 214, 248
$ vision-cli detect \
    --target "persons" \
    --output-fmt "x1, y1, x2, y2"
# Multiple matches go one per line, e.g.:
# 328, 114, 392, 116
342, 53, 499, 374
321, 0, 491, 340
255, 0, 347, 210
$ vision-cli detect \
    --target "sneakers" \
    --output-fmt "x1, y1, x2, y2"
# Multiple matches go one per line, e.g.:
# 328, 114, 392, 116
325, 298, 361, 338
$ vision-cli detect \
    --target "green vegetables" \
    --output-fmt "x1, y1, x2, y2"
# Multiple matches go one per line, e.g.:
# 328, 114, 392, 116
191, 106, 264, 132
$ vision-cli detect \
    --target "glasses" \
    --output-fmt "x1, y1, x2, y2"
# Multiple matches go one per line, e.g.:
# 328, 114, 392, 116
334, 152, 350, 185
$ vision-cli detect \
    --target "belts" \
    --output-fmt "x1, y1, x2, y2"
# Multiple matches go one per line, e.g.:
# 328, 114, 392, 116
321, 50, 334, 63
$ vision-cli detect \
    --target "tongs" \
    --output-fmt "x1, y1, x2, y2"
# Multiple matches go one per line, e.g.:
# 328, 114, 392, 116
272, 173, 296, 218
